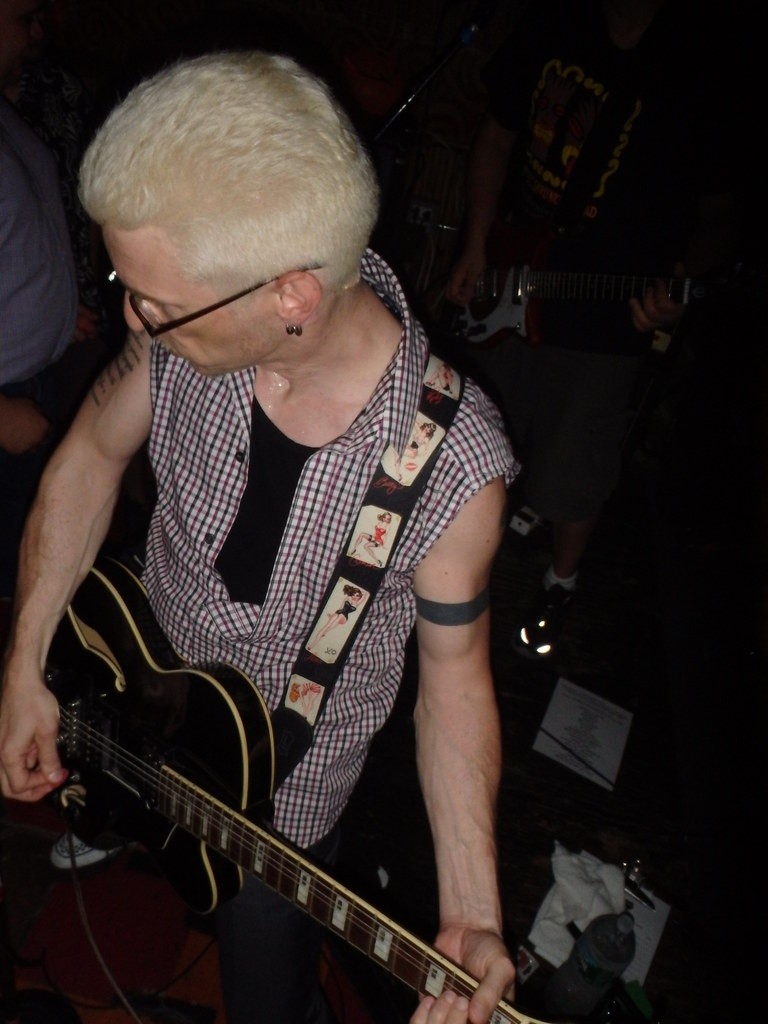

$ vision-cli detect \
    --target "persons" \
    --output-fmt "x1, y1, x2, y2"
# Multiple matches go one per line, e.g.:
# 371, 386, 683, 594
0, 0, 155, 655
444, 0, 730, 641
0, 47, 521, 1024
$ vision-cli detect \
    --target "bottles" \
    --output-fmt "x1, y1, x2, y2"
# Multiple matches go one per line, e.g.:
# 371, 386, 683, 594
549, 913, 636, 1016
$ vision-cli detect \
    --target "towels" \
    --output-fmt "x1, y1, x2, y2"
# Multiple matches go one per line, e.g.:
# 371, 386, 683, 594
539, 839, 624, 936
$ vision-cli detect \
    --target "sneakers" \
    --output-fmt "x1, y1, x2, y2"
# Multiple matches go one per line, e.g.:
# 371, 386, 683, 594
50, 822, 123, 869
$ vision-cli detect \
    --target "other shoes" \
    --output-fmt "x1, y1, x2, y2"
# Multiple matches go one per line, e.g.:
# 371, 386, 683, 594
519, 582, 579, 657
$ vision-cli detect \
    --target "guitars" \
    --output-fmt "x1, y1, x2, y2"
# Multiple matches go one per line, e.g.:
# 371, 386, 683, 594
456, 216, 767, 350
40, 557, 555, 1023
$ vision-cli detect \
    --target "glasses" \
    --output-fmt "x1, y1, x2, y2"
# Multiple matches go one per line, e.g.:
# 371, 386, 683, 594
129, 261, 328, 339
